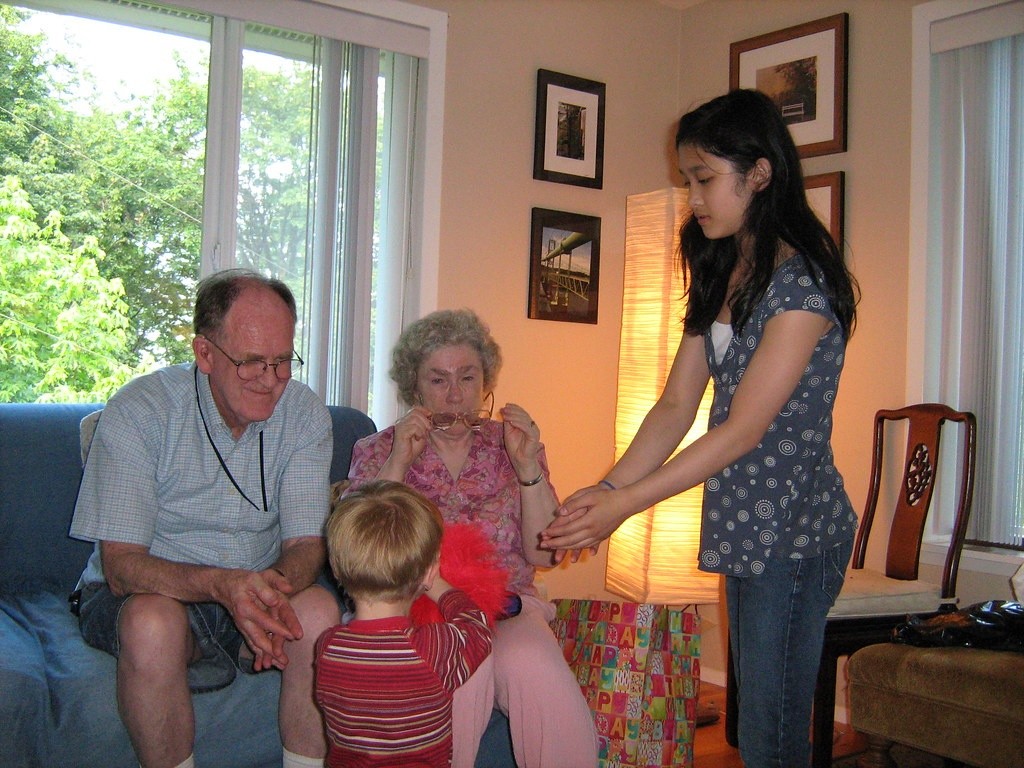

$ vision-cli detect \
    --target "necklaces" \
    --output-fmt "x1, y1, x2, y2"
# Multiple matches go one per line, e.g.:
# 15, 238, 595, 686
194, 366, 269, 513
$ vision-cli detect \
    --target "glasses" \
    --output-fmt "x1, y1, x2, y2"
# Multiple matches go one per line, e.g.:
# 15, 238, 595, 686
418, 391, 494, 430
195, 334, 304, 380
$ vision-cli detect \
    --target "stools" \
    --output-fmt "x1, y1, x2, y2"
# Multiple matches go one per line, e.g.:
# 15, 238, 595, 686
844, 644, 1024, 768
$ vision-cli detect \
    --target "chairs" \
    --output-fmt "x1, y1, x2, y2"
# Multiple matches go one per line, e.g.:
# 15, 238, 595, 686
810, 402, 976, 767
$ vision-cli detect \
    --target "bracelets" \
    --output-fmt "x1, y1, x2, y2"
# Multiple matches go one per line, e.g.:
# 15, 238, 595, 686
598, 480, 615, 490
518, 473, 543, 486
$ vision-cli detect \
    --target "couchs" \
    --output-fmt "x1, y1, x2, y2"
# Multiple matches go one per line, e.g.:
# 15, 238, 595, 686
0, 404, 519, 768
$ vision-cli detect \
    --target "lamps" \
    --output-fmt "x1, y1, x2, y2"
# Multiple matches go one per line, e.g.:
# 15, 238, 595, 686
604, 184, 725, 725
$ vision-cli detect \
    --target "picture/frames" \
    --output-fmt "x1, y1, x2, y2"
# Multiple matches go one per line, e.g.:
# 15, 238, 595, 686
526, 207, 601, 324
728, 12, 850, 162
533, 68, 606, 190
794, 169, 846, 260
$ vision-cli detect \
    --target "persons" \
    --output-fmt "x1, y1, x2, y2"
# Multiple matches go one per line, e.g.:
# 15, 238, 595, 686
66, 274, 341, 768
313, 480, 492, 768
350, 310, 602, 768
540, 90, 857, 768
